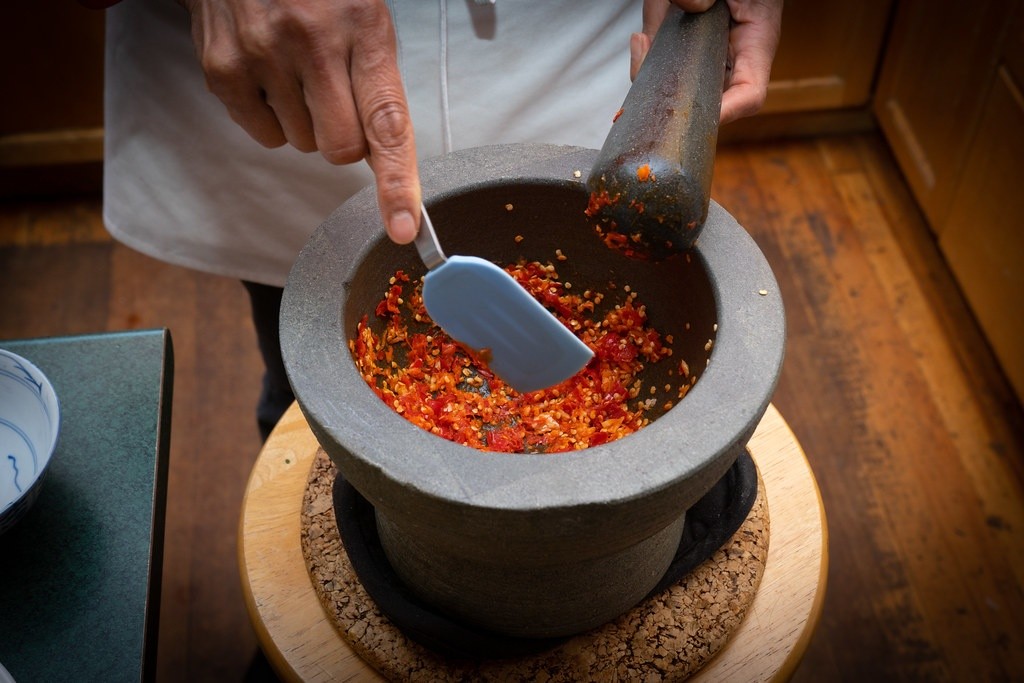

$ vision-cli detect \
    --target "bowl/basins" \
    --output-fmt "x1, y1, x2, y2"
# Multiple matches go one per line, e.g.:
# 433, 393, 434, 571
0, 348, 60, 536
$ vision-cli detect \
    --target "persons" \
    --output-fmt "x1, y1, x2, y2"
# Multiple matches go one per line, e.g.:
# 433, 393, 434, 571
104, 0, 784, 683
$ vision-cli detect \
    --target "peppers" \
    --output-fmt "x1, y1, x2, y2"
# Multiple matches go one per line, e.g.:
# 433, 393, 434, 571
347, 187, 722, 456
